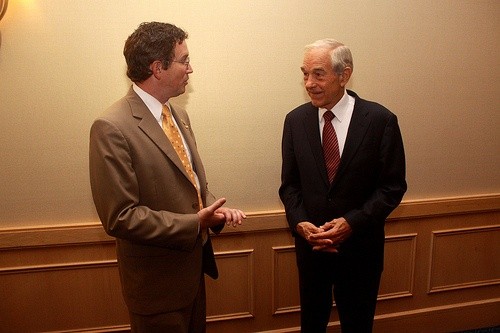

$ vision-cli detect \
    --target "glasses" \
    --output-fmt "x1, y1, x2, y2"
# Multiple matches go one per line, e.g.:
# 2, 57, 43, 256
161, 55, 190, 67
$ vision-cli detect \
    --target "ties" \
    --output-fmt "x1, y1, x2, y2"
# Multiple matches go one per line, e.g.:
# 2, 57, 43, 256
160, 104, 207, 240
322, 111, 341, 194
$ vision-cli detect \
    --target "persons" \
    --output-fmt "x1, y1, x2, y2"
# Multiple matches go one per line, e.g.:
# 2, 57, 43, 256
89, 21, 246, 333
279, 38, 408, 333
